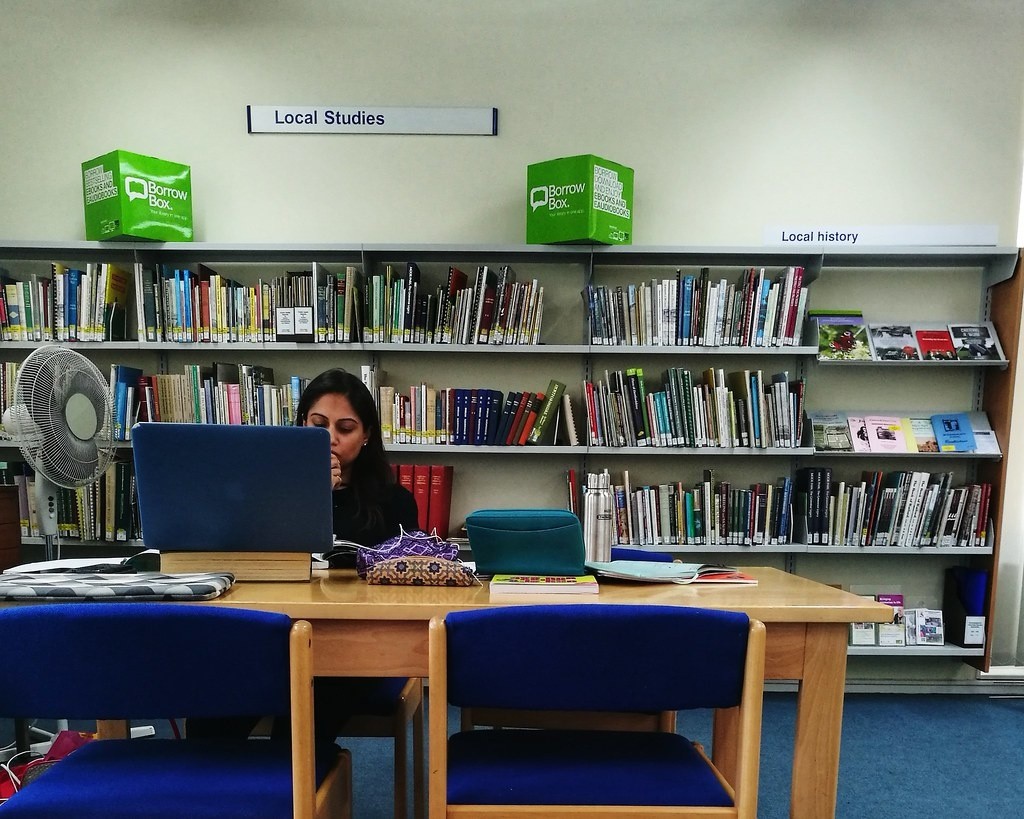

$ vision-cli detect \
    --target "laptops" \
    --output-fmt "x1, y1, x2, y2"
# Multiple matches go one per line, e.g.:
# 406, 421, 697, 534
129, 422, 335, 553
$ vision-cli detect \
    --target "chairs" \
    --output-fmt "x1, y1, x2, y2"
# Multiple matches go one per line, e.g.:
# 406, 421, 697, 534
425, 547, 767, 817
0, 603, 354, 819
250, 676, 425, 818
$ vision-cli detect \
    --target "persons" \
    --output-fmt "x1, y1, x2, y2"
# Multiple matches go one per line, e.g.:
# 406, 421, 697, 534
296, 366, 419, 567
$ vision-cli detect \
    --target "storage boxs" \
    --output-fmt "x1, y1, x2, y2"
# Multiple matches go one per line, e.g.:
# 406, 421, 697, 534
527, 155, 635, 245
82, 149, 193, 243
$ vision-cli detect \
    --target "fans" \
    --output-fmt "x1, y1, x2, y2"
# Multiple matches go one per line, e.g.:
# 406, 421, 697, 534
0, 344, 156, 761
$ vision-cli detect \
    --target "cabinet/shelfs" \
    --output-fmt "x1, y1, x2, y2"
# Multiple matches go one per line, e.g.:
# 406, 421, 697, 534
0, 240, 1024, 698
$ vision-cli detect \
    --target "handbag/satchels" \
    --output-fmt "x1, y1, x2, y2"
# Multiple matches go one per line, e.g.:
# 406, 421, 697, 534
465, 508, 584, 575
356, 531, 458, 579
366, 555, 473, 586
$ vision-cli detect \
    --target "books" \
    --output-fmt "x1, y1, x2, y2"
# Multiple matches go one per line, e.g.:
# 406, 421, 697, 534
952, 564, 988, 617
809, 309, 1008, 361
582, 266, 810, 348
568, 468, 578, 516
134, 261, 361, 343
0, 459, 139, 543
388, 464, 454, 543
805, 408, 1001, 454
0, 361, 22, 440
311, 534, 362, 570
3, 548, 161, 573
101, 363, 136, 442
489, 574, 599, 594
584, 562, 759, 586
0, 262, 133, 344
361, 365, 580, 447
151, 359, 311, 429
604, 467, 793, 546
583, 364, 807, 447
368, 260, 546, 345
904, 607, 945, 646
797, 466, 993, 547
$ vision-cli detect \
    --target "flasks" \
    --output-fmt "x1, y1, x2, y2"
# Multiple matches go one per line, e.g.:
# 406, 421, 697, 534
583, 472, 613, 564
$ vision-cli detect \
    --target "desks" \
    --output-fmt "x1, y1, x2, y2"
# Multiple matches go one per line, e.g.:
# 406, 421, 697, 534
0, 566, 895, 819
